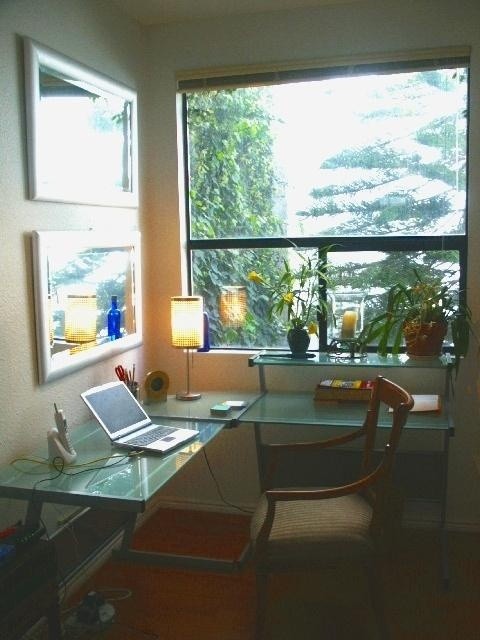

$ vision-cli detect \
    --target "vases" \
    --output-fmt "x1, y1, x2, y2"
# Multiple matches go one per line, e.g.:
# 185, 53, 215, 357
287, 329, 310, 357
402, 319, 444, 362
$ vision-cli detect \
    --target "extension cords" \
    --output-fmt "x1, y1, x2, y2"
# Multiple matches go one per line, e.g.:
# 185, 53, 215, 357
63, 600, 107, 636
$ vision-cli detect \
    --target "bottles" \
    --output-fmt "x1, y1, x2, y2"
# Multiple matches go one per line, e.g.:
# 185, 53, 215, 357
106, 294, 121, 336
196, 311, 210, 352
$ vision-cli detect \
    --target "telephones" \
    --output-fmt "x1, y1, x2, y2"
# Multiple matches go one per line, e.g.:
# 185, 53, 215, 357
47, 403, 77, 463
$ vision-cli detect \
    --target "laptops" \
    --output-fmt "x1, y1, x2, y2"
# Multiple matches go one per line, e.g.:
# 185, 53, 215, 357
80, 379, 202, 456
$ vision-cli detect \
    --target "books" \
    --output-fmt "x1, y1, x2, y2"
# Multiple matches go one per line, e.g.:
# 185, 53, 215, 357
311, 378, 375, 403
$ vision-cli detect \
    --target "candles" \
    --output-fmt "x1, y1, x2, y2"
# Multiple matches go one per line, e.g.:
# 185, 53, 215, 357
342, 310, 356, 339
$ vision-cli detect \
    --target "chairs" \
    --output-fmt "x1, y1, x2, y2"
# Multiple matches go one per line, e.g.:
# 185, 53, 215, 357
248, 374, 413, 638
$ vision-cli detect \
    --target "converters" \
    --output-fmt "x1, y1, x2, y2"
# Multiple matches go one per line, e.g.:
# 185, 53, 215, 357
79, 590, 106, 623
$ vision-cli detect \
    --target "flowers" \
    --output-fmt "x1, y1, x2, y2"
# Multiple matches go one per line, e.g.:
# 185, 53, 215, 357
242, 238, 335, 343
364, 267, 479, 400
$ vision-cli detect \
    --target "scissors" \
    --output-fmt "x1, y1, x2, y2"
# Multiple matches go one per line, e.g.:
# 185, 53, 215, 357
114, 365, 125, 382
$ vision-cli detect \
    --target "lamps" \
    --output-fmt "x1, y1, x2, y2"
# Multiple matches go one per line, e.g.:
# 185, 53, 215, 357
169, 296, 204, 400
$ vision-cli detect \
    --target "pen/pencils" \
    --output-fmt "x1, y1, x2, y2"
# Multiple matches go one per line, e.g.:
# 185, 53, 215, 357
125, 365, 137, 382
